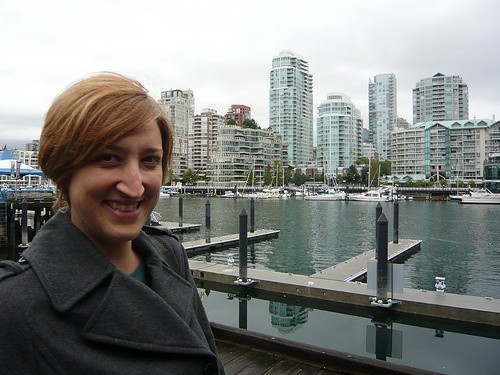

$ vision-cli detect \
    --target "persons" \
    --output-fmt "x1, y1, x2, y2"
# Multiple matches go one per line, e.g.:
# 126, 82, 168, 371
0, 71, 227, 375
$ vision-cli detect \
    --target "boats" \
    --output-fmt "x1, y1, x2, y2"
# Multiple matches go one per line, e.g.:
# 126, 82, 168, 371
458, 187, 500, 204
448, 191, 474, 201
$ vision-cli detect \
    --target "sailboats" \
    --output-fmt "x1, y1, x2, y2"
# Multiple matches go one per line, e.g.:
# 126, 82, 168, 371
220, 155, 416, 203
